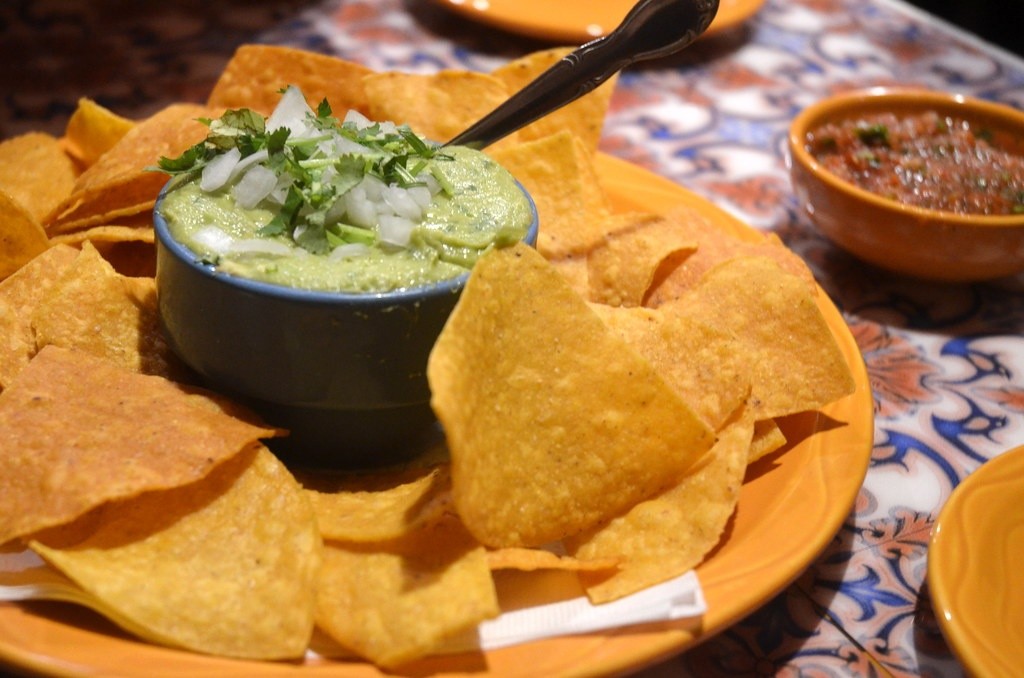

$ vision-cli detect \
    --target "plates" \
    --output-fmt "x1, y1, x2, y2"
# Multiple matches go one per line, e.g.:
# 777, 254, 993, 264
927, 444, 1024, 678
435, 0, 766, 43
0, 146, 874, 678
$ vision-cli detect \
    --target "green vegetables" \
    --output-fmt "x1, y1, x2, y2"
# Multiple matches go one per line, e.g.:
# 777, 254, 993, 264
142, 86, 457, 252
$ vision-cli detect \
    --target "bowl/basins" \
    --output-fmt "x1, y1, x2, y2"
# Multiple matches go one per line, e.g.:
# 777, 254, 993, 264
154, 139, 540, 471
787, 88, 1024, 284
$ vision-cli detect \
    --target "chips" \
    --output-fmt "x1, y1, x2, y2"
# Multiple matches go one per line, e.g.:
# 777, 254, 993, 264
0, 44, 860, 668
